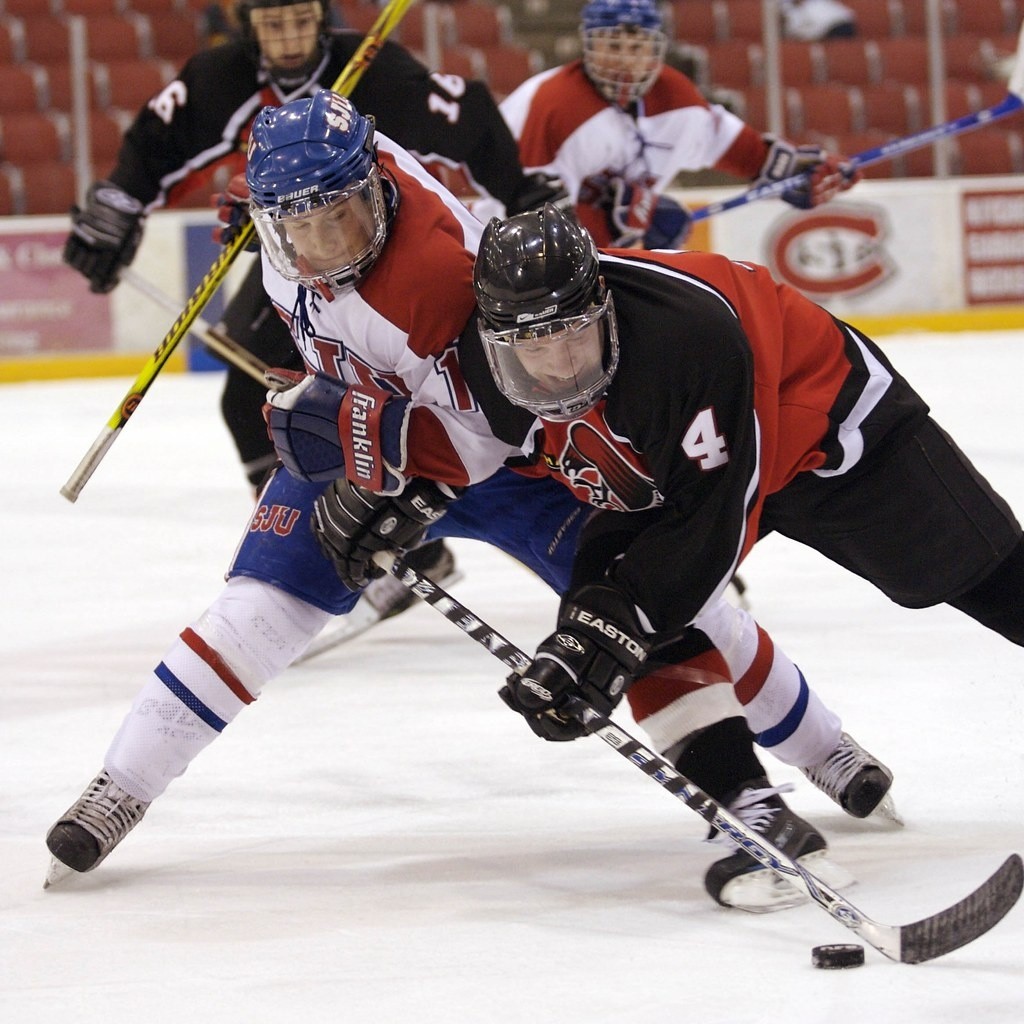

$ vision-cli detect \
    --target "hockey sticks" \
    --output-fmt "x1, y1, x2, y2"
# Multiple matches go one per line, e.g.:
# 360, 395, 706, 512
116, 255, 278, 387
369, 549, 1024, 964
691, 95, 1022, 222
56, 1, 409, 505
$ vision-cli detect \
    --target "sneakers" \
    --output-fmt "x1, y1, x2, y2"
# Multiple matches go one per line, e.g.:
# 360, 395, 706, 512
369, 546, 455, 616
42, 766, 152, 888
798, 732, 907, 826
704, 783, 857, 912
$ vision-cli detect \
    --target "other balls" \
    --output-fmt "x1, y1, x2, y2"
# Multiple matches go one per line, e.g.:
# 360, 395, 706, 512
810, 942, 865, 971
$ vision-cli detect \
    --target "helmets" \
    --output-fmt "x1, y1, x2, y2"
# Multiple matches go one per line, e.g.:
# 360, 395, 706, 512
237, 0, 330, 77
581, 0, 670, 103
472, 202, 601, 339
245, 88, 376, 215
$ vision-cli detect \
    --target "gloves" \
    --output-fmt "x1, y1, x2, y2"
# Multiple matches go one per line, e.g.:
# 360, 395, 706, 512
61, 182, 148, 294
309, 473, 459, 596
608, 177, 693, 249
497, 580, 656, 743
262, 368, 414, 497
749, 131, 862, 209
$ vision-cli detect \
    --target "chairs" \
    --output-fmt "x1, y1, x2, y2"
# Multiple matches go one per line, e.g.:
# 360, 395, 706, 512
0, 1, 1023, 216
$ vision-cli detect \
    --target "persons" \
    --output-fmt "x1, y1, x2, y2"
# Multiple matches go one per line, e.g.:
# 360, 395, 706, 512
310, 204, 1024, 908
497, 0, 863, 248
45, 89, 895, 874
64, 0, 555, 619
